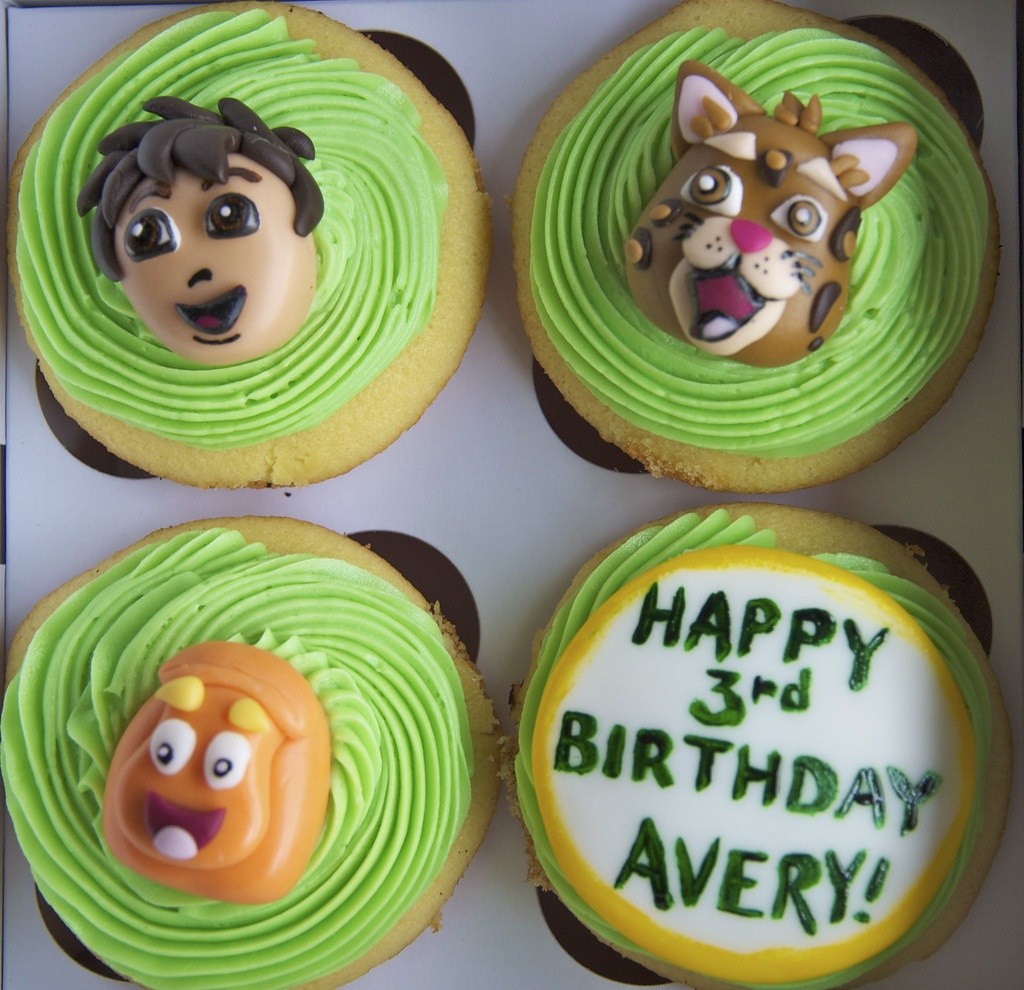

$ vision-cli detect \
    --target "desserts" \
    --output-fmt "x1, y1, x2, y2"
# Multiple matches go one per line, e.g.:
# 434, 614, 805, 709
3, 2, 1006, 990
